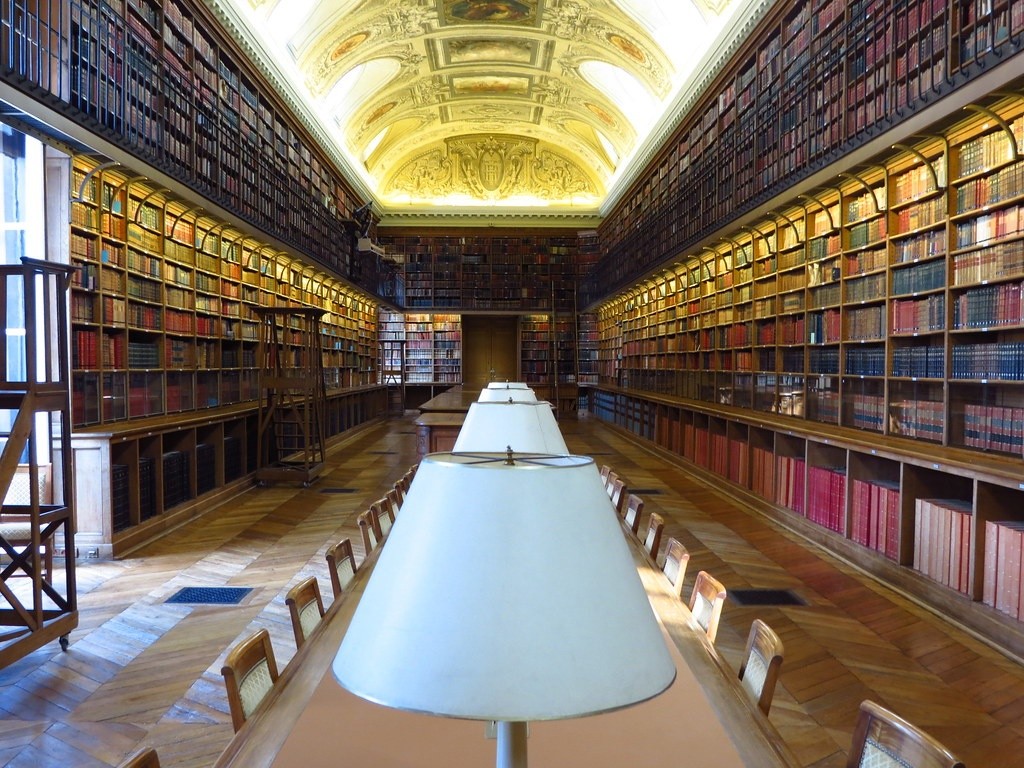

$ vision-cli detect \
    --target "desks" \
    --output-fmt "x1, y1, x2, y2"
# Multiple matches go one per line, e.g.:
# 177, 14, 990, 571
204, 524, 804, 768
447, 385, 486, 392
418, 392, 480, 412
415, 413, 466, 460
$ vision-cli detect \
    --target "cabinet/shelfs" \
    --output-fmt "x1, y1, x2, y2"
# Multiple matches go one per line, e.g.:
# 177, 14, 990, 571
0, 0, 1024, 619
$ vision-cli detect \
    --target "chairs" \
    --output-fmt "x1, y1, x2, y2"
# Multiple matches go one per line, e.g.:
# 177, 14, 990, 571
610, 480, 626, 512
115, 464, 420, 768
605, 472, 620, 498
624, 494, 644, 533
738, 616, 784, 716
661, 538, 690, 594
688, 570, 727, 643
844, 698, 961, 768
644, 513, 665, 559
601, 465, 611, 487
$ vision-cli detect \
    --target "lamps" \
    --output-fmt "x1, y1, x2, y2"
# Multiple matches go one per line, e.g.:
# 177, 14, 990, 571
450, 396, 574, 457
328, 446, 678, 768
477, 385, 538, 403
488, 379, 528, 389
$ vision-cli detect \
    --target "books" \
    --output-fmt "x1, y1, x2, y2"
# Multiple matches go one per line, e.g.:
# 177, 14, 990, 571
0, 0, 1024, 625
